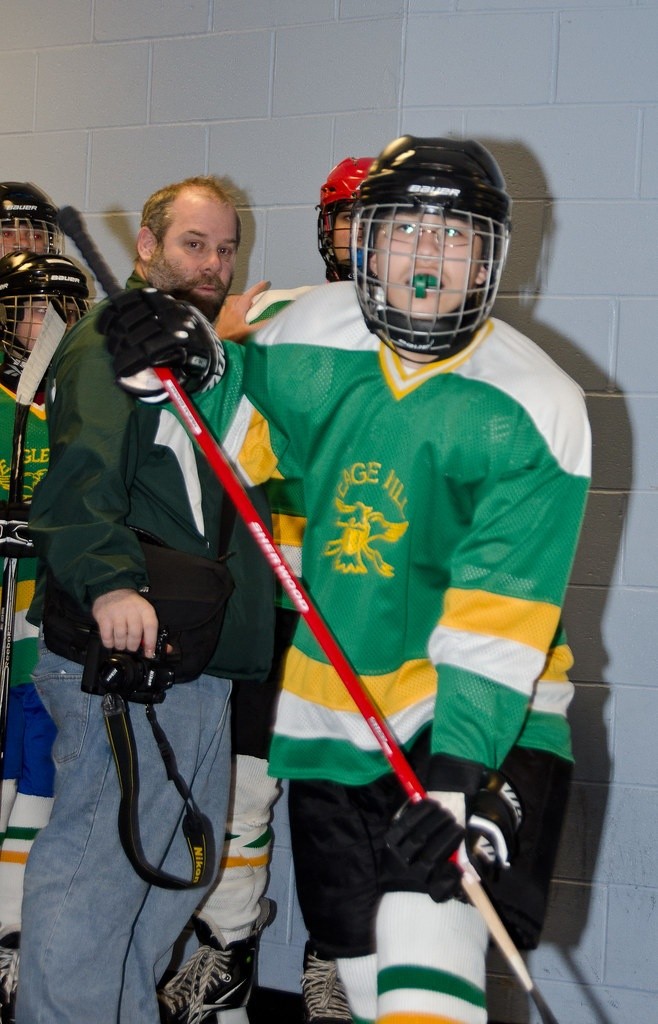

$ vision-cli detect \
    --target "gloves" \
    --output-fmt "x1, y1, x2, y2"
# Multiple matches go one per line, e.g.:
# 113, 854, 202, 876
383, 754, 522, 902
93, 287, 228, 405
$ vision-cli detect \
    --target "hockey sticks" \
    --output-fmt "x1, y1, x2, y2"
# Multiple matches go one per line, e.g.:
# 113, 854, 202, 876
0, 300, 69, 757
53, 200, 562, 1023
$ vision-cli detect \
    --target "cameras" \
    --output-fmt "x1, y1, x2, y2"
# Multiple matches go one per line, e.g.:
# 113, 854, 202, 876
81, 641, 175, 704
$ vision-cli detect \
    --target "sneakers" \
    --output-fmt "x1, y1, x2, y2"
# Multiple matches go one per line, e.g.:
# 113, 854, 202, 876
157, 897, 277, 1024
0, 921, 22, 1024
301, 940, 355, 1024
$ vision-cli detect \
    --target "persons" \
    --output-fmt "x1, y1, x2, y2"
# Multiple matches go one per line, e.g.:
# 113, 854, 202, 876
95, 133, 591, 1023
157, 157, 378, 1023
15, 177, 277, 1024
0, 179, 90, 1024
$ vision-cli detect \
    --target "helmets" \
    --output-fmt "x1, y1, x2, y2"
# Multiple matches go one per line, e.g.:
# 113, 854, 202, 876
315, 157, 376, 281
1, 181, 89, 368
351, 136, 511, 363
